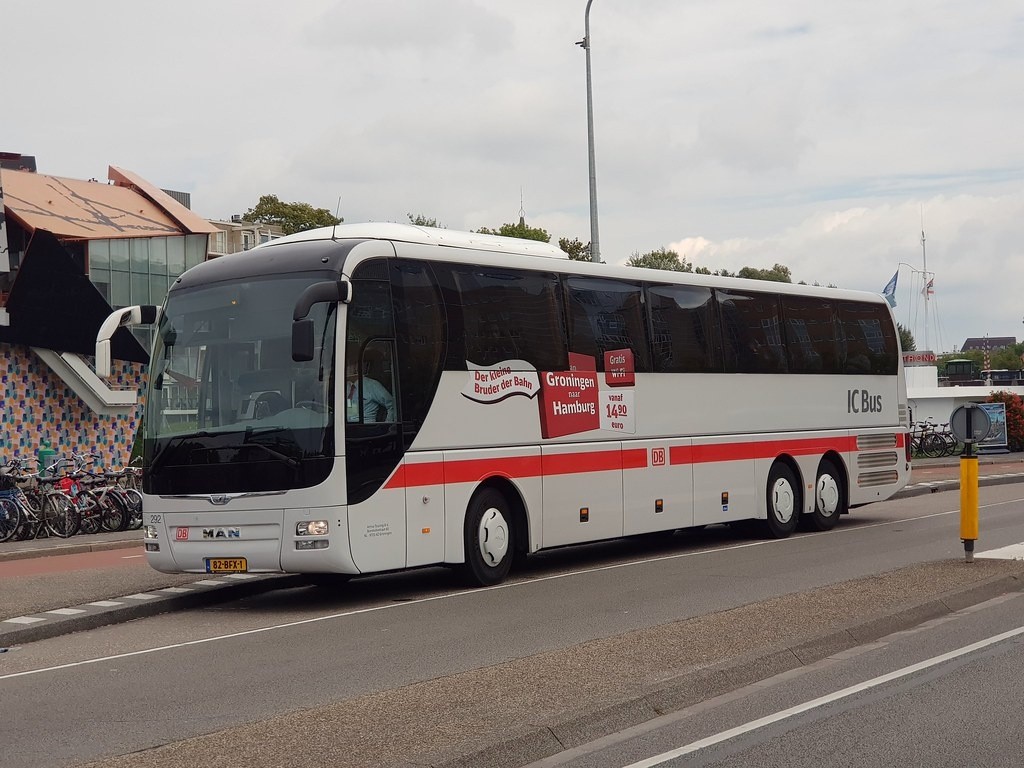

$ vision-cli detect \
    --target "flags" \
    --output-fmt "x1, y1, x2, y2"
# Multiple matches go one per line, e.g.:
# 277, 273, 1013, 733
882, 271, 897, 309
922, 279, 934, 299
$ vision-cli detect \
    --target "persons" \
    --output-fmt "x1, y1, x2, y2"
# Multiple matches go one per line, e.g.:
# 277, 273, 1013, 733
340, 346, 395, 420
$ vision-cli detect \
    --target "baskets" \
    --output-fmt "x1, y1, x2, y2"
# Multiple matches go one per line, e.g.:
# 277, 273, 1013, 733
0, 475, 15, 491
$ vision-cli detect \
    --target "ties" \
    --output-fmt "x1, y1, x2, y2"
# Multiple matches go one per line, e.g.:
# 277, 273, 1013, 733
348, 384, 356, 400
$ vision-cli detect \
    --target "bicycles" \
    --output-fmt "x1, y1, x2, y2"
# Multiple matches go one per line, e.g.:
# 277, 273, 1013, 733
911, 416, 965, 459
0, 452, 142, 543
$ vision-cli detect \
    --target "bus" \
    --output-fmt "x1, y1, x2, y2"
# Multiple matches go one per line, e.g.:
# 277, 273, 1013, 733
95, 221, 912, 589
